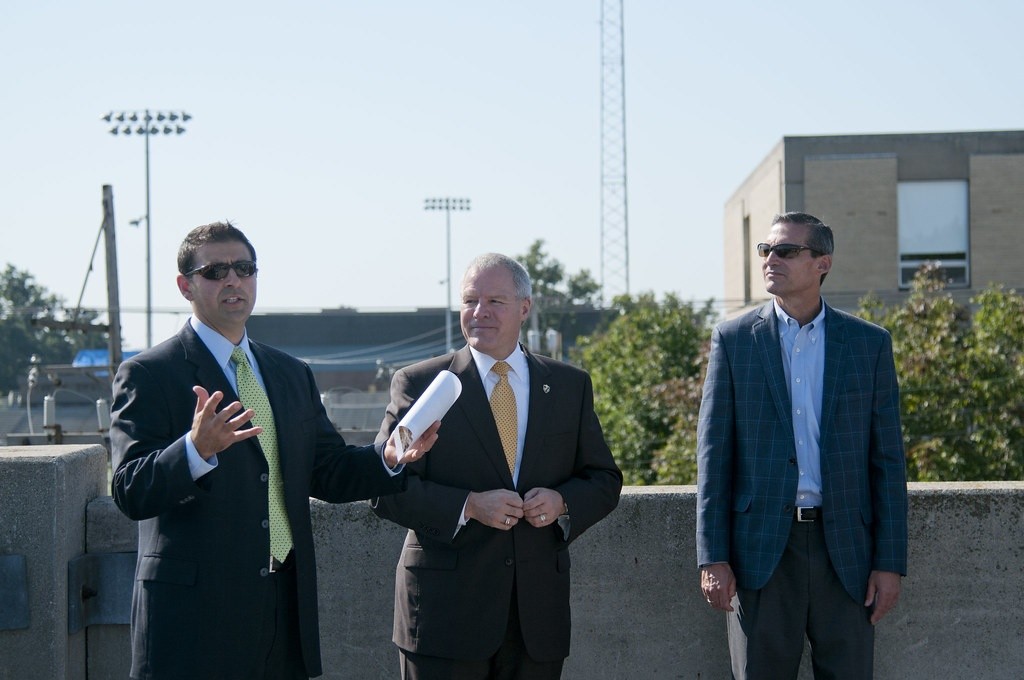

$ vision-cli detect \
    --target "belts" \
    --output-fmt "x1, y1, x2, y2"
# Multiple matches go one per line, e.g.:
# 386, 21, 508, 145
269, 554, 296, 572
796, 506, 822, 520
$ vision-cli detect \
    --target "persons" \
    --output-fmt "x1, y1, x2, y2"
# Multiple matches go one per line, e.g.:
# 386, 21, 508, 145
369, 251, 625, 680
109, 219, 443, 679
693, 210, 910, 680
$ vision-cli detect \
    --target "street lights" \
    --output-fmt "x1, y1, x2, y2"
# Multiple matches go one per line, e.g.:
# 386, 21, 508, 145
98, 109, 192, 348
423, 197, 471, 355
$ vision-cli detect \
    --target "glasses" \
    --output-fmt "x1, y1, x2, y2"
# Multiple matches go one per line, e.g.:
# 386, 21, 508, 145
183, 261, 258, 280
755, 243, 825, 259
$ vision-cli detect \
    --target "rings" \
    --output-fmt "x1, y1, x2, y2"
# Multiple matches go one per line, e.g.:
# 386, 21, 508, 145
504, 516, 510, 526
539, 515, 547, 523
420, 441, 429, 454
706, 599, 715, 603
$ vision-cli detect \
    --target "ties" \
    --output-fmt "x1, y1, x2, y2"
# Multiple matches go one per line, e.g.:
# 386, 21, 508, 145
490, 360, 518, 479
232, 346, 295, 562
